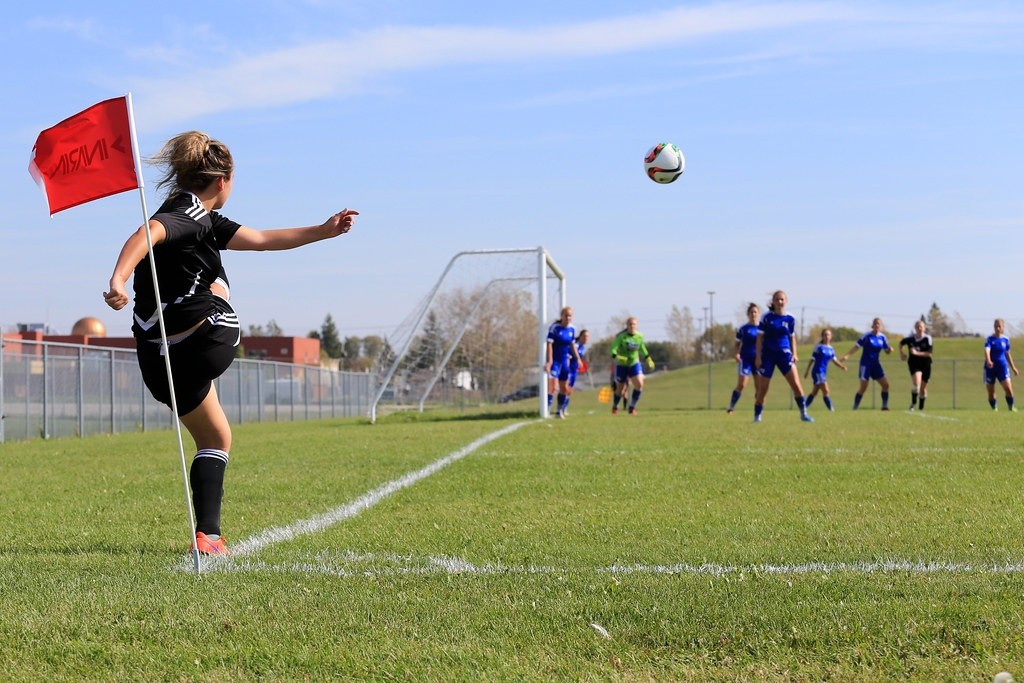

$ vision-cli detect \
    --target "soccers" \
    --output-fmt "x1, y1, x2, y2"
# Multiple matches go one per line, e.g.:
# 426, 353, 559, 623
643, 141, 687, 185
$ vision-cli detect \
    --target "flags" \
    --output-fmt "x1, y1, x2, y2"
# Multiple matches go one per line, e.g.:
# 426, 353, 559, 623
28, 96, 140, 219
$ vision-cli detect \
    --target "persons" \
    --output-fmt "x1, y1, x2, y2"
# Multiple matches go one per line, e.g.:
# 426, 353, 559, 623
984, 318, 1019, 412
544, 307, 583, 419
610, 358, 632, 411
804, 329, 848, 412
840, 317, 894, 411
899, 320, 933, 411
754, 291, 815, 423
558, 330, 590, 416
610, 316, 654, 416
727, 304, 763, 414
103, 131, 359, 555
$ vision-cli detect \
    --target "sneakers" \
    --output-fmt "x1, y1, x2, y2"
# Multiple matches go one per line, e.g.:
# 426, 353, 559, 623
992, 408, 998, 412
909, 405, 916, 411
191, 532, 232, 555
629, 409, 636, 416
556, 411, 566, 420
1009, 408, 1018, 412
800, 416, 814, 422
612, 408, 617, 414
727, 409, 733, 414
563, 408, 568, 416
755, 415, 761, 422
